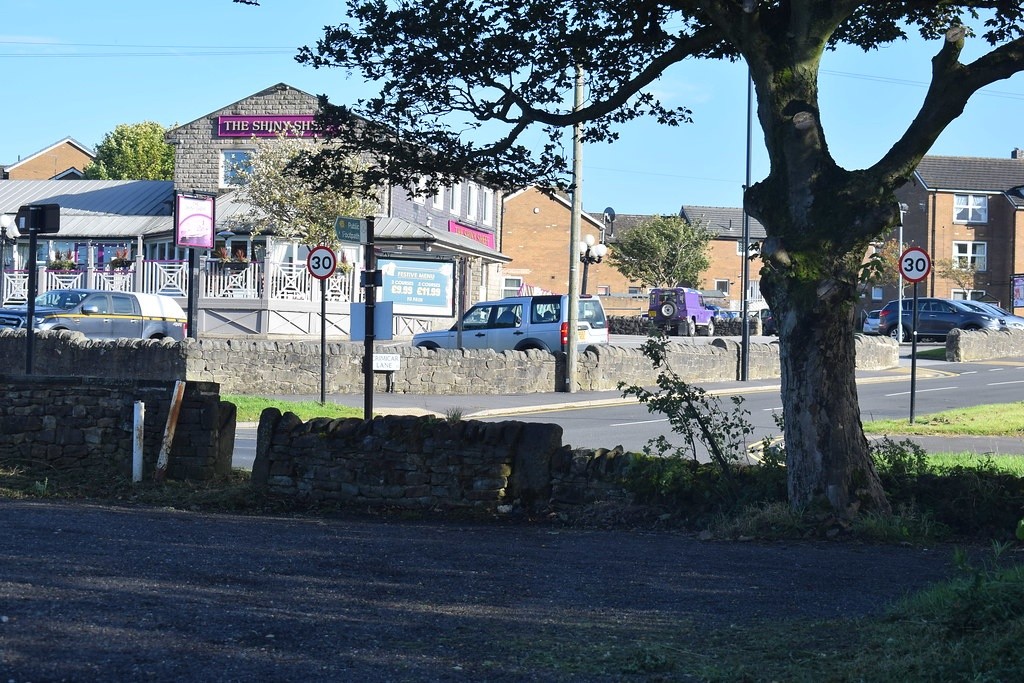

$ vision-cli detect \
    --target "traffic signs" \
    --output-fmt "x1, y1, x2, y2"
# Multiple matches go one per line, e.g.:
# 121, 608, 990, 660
334, 215, 368, 245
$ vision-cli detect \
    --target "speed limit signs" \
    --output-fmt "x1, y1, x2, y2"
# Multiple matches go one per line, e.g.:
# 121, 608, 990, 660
898, 247, 931, 283
307, 246, 336, 280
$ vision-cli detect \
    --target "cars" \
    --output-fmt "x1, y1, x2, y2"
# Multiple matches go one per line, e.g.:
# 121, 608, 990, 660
705, 303, 736, 319
863, 309, 884, 336
752, 309, 780, 337
958, 299, 1024, 329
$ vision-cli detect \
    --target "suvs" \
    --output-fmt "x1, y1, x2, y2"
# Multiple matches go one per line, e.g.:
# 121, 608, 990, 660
878, 297, 1000, 344
648, 287, 715, 337
411, 293, 609, 356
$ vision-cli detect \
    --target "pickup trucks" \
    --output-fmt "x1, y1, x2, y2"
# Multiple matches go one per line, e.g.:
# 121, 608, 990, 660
0, 288, 188, 343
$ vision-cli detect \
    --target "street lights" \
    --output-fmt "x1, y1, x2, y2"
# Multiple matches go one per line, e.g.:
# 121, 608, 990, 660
579, 234, 608, 294
897, 202, 910, 345
